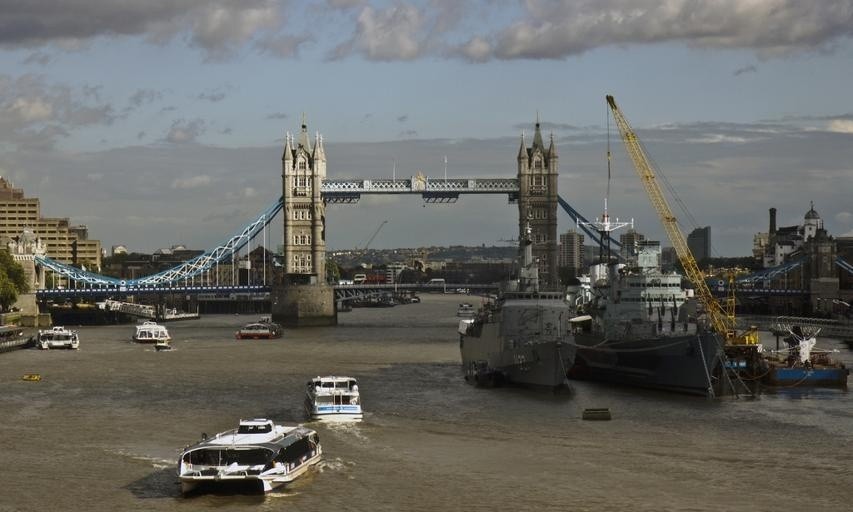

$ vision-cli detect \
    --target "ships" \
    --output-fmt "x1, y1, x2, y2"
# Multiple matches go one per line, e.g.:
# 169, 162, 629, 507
558, 197, 730, 401
454, 207, 582, 392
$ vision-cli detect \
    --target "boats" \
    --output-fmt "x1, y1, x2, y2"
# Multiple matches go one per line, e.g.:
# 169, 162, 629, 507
173, 417, 327, 495
457, 302, 475, 317
132, 320, 174, 344
302, 373, 363, 424
756, 323, 852, 388
152, 337, 171, 352
234, 316, 284, 339
36, 326, 80, 351
